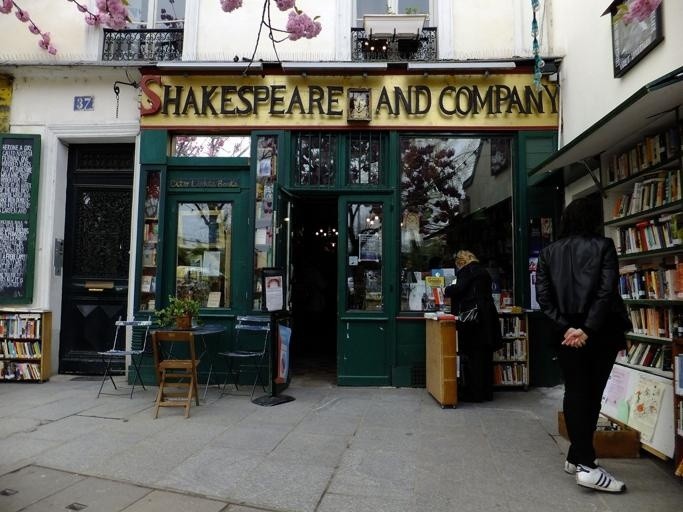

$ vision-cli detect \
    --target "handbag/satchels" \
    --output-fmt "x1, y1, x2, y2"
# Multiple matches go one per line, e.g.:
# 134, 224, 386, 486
457, 308, 480, 333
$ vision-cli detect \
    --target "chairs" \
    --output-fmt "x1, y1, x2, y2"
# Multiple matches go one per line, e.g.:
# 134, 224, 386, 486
216, 315, 272, 401
149, 329, 201, 418
96, 316, 153, 400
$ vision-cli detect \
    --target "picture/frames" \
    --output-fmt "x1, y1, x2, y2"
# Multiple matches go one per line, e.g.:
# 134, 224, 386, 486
610, 0, 663, 77
491, 135, 508, 177
348, 88, 372, 123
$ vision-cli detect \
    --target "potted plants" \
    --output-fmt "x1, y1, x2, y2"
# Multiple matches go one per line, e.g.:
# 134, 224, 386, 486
154, 287, 201, 330
363, 0, 429, 40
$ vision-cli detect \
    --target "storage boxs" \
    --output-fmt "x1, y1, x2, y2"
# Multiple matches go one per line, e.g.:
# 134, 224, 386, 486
558, 412, 641, 459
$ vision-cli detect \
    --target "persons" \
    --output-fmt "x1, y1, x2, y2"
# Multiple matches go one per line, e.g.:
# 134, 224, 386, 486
535, 196, 629, 493
444, 249, 505, 403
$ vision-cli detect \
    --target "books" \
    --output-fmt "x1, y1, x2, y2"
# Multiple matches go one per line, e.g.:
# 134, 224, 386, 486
423, 313, 528, 386
596, 122, 683, 375
0, 313, 41, 380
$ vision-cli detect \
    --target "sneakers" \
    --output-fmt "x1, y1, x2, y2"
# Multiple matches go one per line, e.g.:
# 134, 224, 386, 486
564, 459, 628, 493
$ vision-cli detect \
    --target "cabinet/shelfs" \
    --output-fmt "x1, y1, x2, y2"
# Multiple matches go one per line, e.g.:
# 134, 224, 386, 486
598, 126, 682, 482
425, 309, 532, 409
1, 307, 54, 384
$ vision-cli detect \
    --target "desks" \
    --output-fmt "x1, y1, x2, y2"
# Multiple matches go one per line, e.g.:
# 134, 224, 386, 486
148, 322, 228, 403
